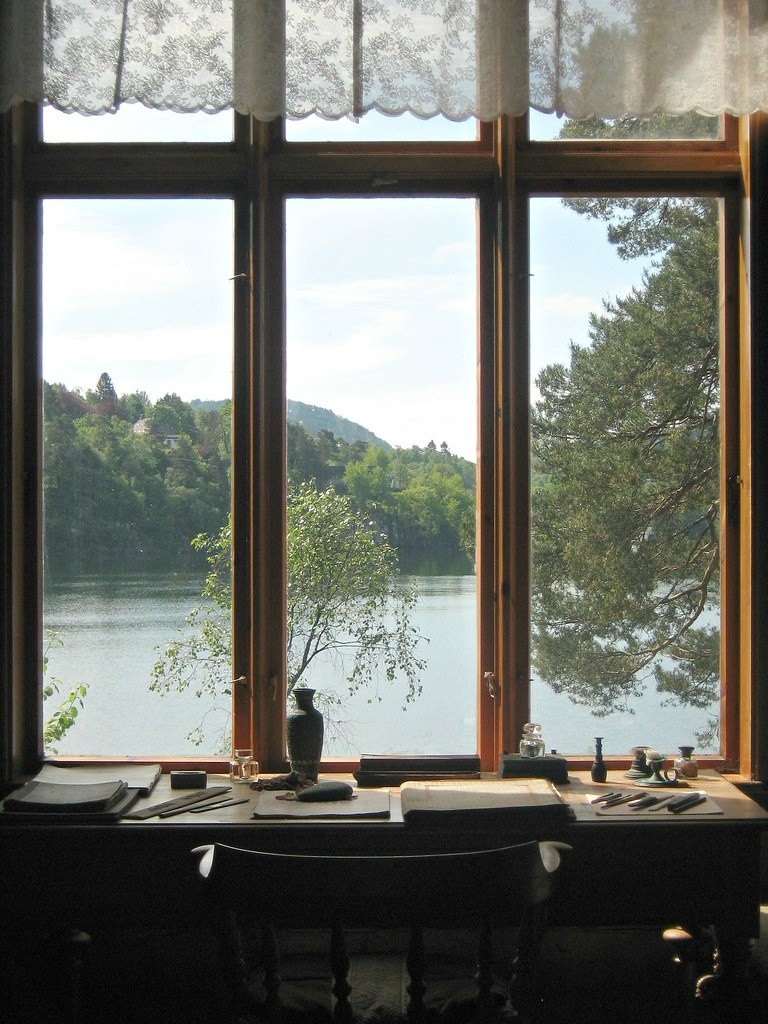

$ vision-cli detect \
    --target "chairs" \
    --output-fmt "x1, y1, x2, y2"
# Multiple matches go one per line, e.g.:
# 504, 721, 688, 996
193, 840, 557, 1024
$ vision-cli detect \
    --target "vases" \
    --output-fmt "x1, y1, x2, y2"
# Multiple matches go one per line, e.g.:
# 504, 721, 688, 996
287, 689, 323, 783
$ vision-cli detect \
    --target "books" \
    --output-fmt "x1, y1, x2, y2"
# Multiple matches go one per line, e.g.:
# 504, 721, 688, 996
353, 754, 481, 787
250, 789, 389, 820
0, 781, 141, 821
25, 763, 163, 794
401, 778, 577, 822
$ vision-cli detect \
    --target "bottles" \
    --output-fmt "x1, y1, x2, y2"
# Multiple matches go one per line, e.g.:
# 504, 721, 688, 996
591, 737, 607, 782
674, 746, 698, 780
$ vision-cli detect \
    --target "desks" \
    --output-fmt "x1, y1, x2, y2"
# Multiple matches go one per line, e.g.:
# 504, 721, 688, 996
0, 774, 768, 1024
